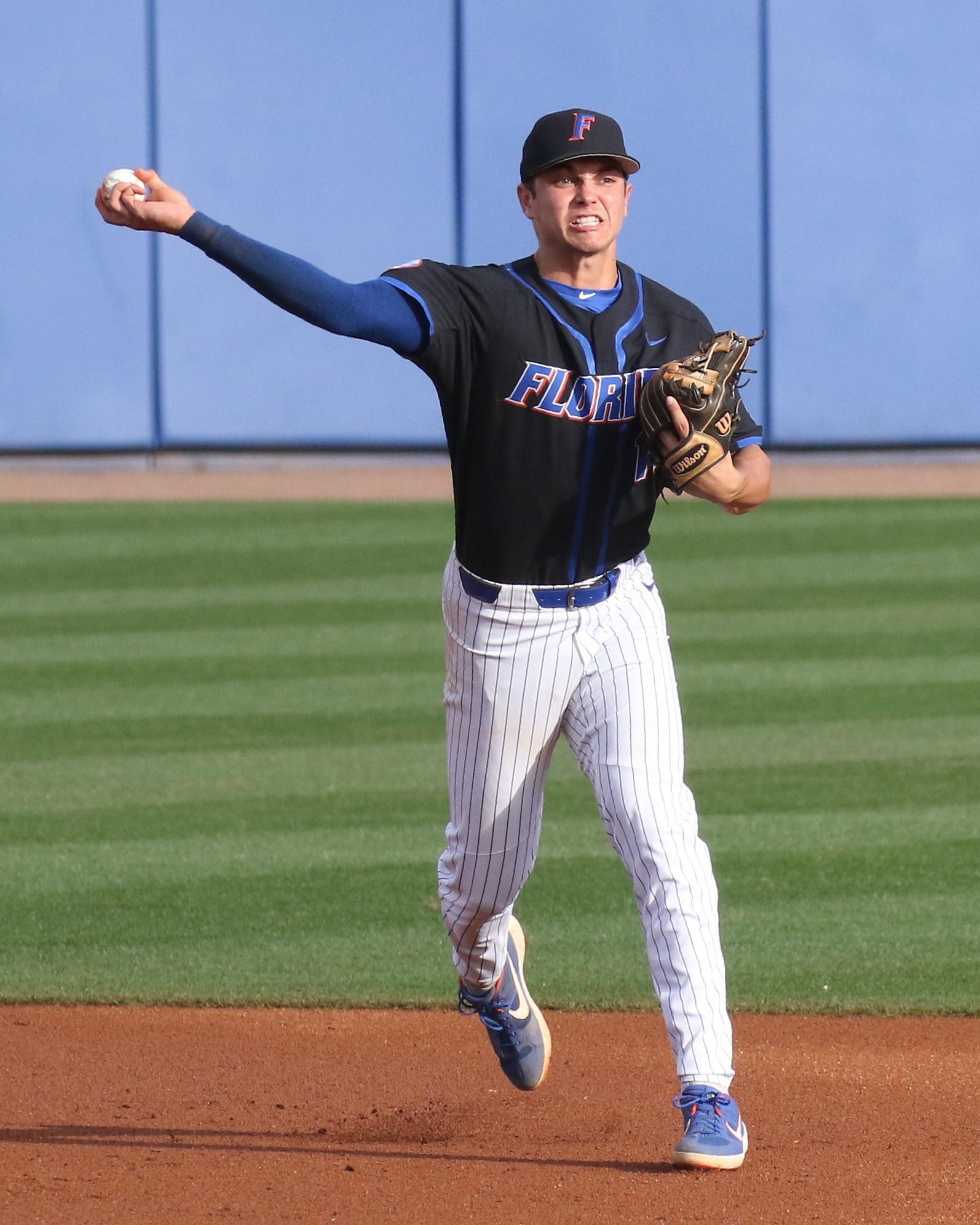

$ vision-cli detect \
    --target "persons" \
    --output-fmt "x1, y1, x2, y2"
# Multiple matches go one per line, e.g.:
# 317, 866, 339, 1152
93, 107, 772, 1168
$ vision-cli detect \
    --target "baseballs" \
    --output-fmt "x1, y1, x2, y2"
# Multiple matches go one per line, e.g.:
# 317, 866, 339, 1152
101, 168, 146, 205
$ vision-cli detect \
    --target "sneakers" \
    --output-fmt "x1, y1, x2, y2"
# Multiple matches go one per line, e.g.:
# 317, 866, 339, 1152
672, 1085, 748, 1169
457, 914, 552, 1091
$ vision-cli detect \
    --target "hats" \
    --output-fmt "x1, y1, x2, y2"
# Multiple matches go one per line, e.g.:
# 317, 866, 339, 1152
520, 108, 640, 181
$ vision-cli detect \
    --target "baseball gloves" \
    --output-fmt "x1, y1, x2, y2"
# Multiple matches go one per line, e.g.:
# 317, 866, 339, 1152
637, 328, 751, 489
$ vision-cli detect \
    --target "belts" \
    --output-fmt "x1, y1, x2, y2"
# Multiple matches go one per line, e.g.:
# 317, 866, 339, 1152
458, 566, 621, 611
